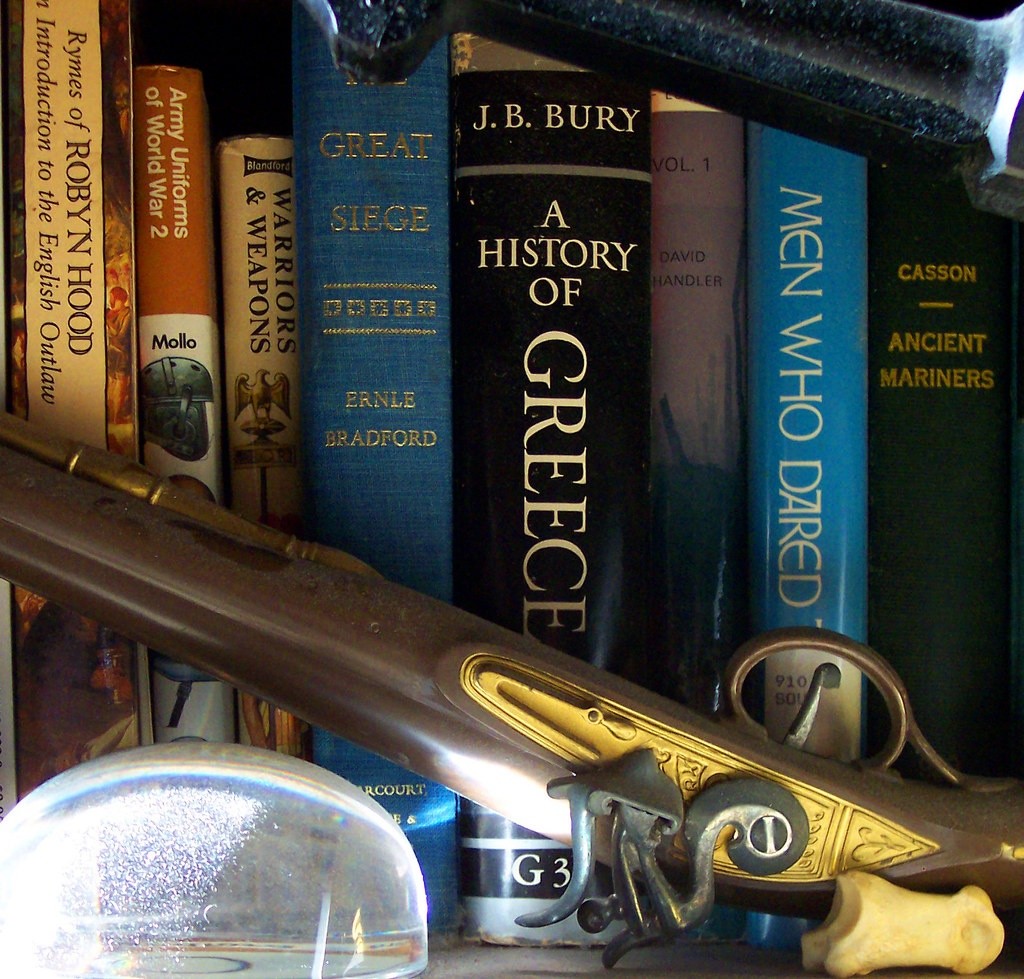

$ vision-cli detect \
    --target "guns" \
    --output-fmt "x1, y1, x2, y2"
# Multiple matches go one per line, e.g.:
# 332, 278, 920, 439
0, 409, 1017, 924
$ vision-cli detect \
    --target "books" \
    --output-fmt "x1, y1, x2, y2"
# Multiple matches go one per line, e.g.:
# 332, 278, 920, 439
0, 0, 1024, 944
